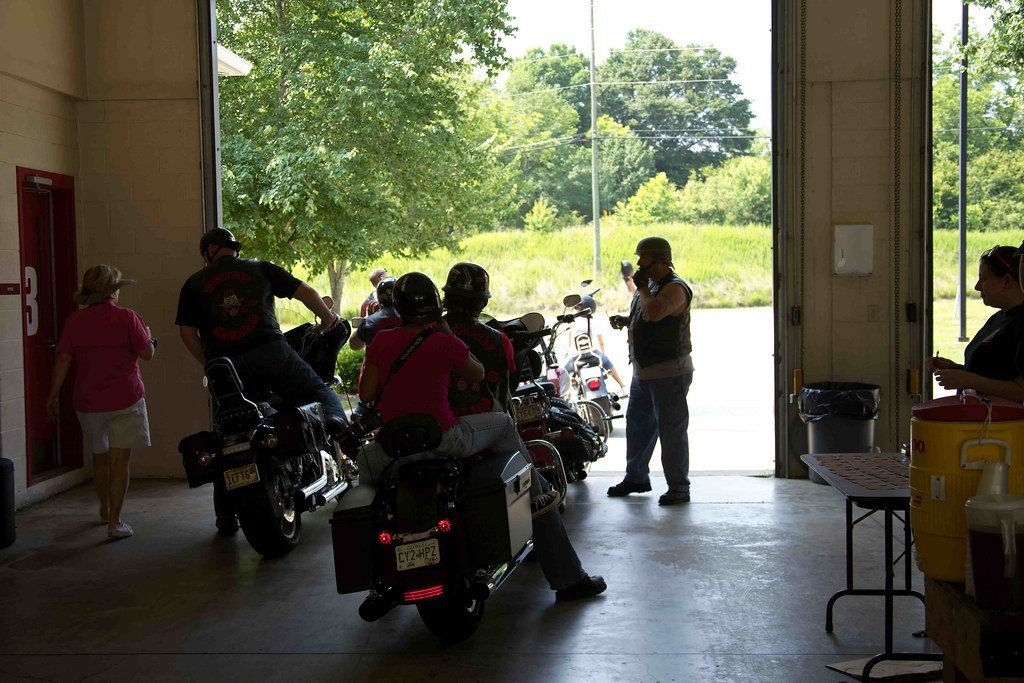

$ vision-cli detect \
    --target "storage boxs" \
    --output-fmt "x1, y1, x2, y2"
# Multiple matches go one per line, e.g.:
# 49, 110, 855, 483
176, 430, 222, 490
456, 451, 533, 566
924, 579, 1024, 683
268, 400, 325, 462
328, 484, 389, 596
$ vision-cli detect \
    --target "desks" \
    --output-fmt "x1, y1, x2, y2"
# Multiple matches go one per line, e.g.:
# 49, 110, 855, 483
799, 452, 943, 683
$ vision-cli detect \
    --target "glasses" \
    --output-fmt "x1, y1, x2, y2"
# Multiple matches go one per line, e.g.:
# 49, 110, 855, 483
376, 268, 388, 280
986, 245, 1015, 276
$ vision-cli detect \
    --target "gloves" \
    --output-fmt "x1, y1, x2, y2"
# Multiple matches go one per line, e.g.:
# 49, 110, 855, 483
633, 270, 650, 291
608, 314, 628, 329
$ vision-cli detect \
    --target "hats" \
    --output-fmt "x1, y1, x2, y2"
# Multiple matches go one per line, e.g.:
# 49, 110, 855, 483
73, 264, 135, 305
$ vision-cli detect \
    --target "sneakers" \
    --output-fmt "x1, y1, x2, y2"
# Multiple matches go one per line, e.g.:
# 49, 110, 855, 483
107, 521, 133, 537
659, 490, 691, 504
531, 490, 560, 519
607, 481, 651, 496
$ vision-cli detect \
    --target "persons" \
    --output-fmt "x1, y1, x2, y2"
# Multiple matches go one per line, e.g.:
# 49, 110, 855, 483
606, 237, 693, 504
624, 275, 636, 294
349, 276, 401, 350
357, 272, 561, 518
926, 239, 1024, 405
46, 263, 157, 536
360, 268, 387, 317
174, 227, 360, 535
442, 263, 608, 602
564, 295, 630, 394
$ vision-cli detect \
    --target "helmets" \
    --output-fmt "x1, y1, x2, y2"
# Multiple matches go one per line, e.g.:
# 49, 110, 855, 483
636, 237, 672, 265
199, 228, 241, 258
441, 263, 491, 299
575, 294, 597, 316
391, 272, 443, 323
376, 279, 395, 306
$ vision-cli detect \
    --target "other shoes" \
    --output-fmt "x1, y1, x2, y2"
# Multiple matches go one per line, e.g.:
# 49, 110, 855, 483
324, 414, 362, 461
215, 515, 239, 533
556, 574, 607, 602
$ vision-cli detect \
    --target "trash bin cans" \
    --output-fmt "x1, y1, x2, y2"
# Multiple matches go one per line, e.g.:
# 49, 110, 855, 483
801, 382, 880, 486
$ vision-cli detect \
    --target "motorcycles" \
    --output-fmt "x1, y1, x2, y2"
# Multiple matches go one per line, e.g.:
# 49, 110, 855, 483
328, 294, 582, 649
177, 295, 359, 560
519, 280, 622, 514
